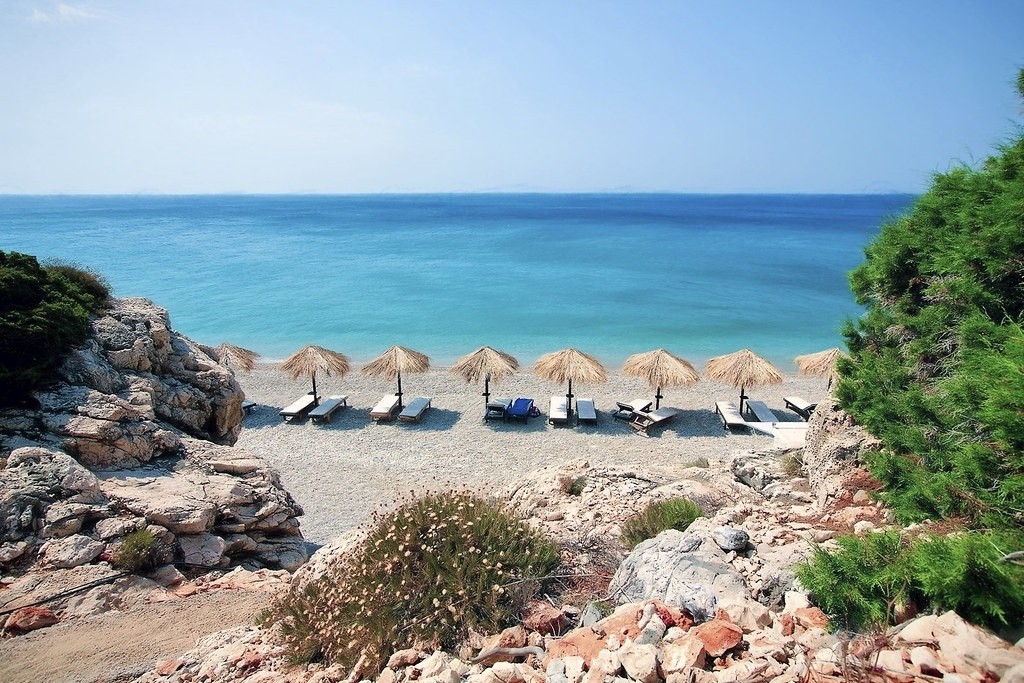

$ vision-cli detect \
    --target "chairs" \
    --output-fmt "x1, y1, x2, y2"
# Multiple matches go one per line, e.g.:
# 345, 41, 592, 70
611, 398, 652, 421
397, 398, 432, 421
370, 395, 399, 421
630, 409, 678, 430
782, 396, 818, 413
278, 395, 320, 421
548, 395, 568, 425
746, 400, 778, 422
483, 398, 511, 423
308, 395, 348, 421
507, 399, 534, 425
575, 398, 596, 427
715, 401, 746, 429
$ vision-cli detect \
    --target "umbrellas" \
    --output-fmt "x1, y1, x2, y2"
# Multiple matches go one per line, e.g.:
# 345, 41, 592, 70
706, 349, 782, 416
215, 343, 256, 370
531, 348, 607, 416
361, 344, 429, 412
624, 349, 701, 410
796, 348, 850, 390
450, 346, 519, 408
282, 345, 350, 408
